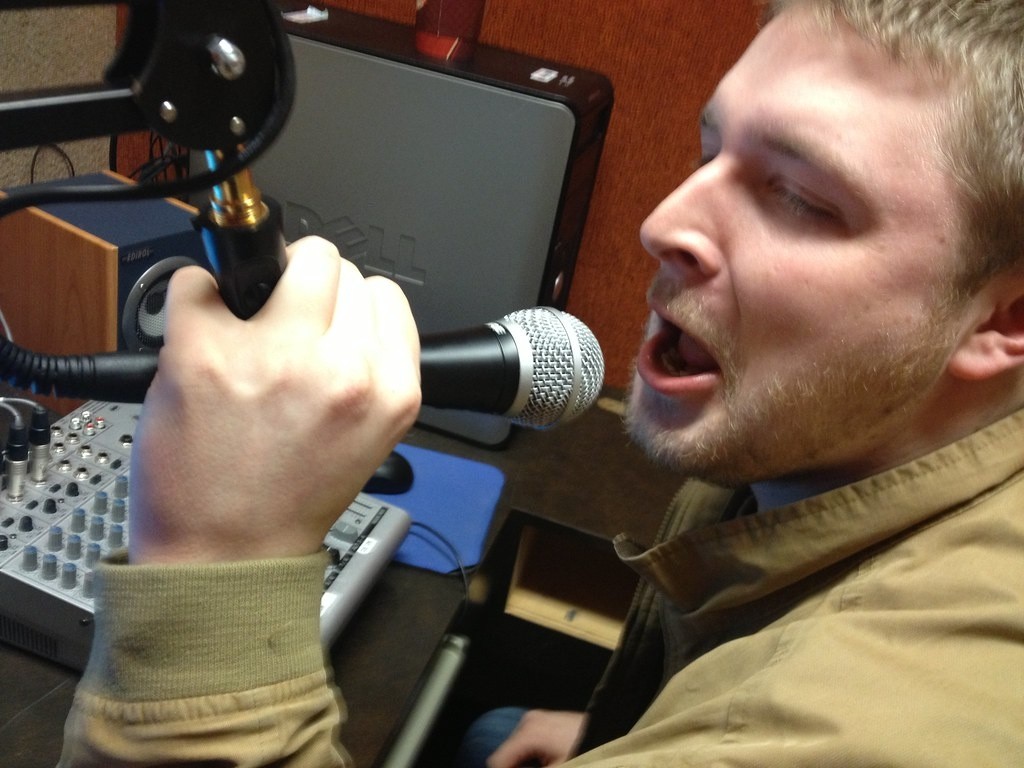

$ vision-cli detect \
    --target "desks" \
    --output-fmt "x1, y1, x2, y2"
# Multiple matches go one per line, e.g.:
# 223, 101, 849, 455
1, 384, 682, 766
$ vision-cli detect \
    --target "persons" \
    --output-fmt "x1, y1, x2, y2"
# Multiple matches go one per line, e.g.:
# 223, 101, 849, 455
54, 0, 1024, 765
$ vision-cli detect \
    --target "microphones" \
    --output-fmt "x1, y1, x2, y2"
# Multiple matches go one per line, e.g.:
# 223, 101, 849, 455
95, 306, 606, 434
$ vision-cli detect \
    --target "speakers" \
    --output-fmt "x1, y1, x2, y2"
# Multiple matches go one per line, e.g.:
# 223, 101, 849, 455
0, 168, 222, 355
